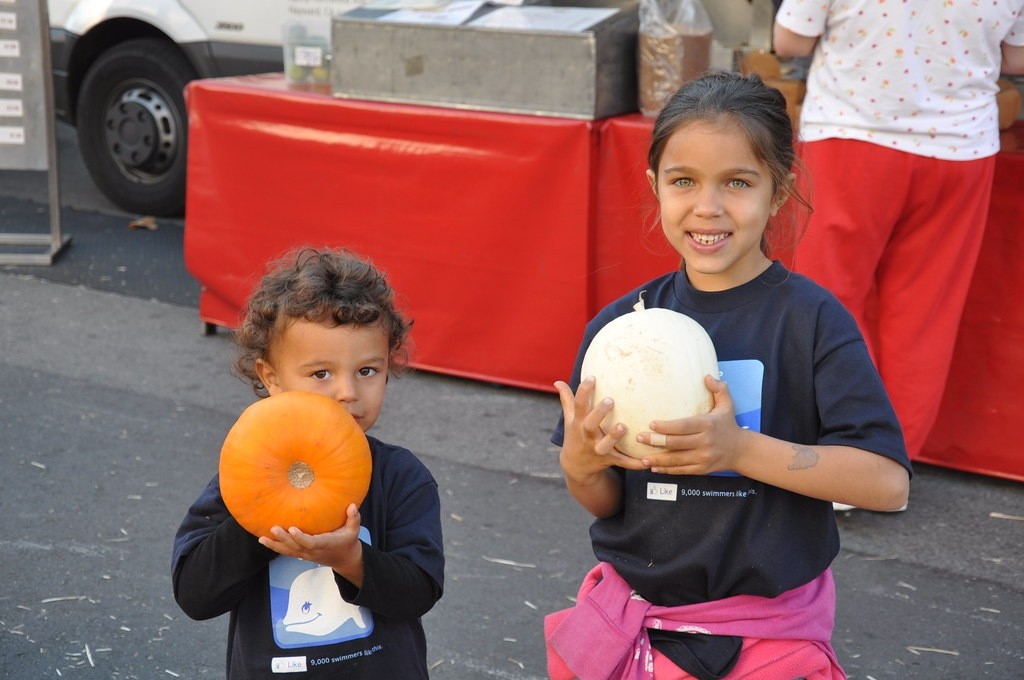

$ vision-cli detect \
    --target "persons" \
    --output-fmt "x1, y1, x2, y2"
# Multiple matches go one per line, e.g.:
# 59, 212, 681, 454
169, 246, 446, 680
773, 0, 1024, 511
543, 70, 914, 680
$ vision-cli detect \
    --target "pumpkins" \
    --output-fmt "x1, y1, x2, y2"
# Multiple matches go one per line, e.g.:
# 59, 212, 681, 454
581, 309, 719, 460
218, 391, 373, 541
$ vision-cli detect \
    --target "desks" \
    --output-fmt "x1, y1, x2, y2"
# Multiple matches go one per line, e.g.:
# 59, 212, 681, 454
595, 109, 1024, 484
181, 61, 640, 395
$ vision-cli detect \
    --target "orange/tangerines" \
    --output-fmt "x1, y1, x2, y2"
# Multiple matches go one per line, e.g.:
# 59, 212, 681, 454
312, 66, 332, 83
289, 66, 307, 82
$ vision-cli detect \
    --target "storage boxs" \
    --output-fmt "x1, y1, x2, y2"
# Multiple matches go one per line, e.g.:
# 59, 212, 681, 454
331, 0, 642, 120
281, 17, 331, 85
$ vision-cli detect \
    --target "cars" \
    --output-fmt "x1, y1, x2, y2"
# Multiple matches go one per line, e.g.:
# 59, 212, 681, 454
47, 0, 524, 214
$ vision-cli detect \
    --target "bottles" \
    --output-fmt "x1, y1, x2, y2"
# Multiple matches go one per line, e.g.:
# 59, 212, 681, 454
665, 1, 714, 84
